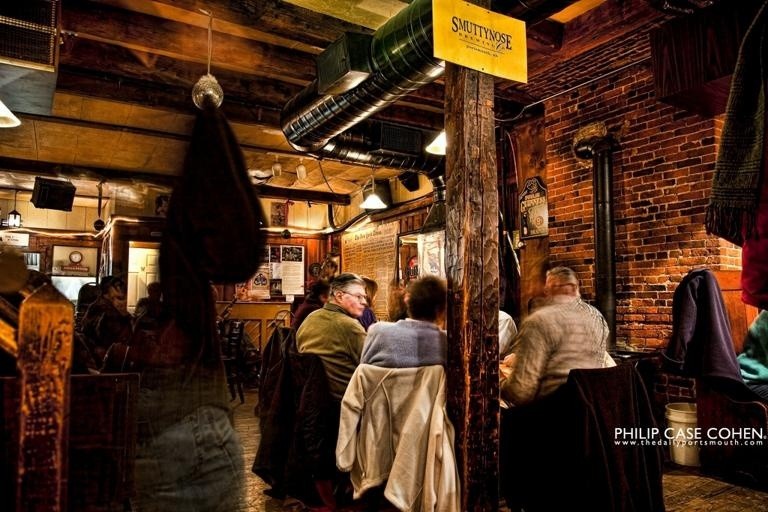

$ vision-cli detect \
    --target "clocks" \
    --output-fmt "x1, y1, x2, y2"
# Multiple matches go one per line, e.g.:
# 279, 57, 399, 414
69, 251, 83, 267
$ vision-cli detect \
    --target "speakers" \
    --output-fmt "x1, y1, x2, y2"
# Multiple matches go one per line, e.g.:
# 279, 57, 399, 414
30, 176, 76, 211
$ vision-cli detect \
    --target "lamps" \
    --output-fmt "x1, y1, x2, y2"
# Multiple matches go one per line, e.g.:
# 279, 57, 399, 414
424, 127, 447, 156
192, 9, 223, 110
360, 167, 386, 210
7, 189, 21, 228
0, 100, 23, 129
271, 153, 307, 182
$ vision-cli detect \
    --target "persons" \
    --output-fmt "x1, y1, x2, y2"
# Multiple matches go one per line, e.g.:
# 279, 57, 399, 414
499, 291, 616, 371
1, 242, 518, 512
496, 265, 619, 511
734, 306, 768, 407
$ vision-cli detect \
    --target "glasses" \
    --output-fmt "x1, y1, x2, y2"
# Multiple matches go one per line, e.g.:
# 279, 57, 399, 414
339, 289, 368, 300
